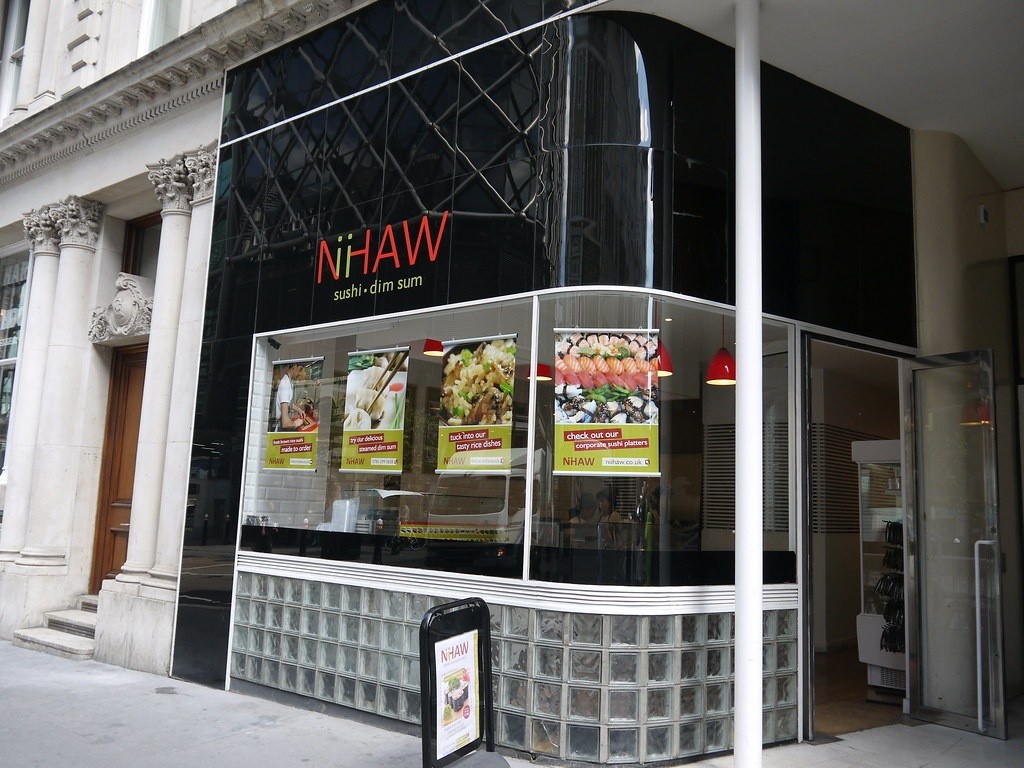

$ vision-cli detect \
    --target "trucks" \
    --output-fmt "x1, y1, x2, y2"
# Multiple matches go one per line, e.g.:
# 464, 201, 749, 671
428, 449, 549, 546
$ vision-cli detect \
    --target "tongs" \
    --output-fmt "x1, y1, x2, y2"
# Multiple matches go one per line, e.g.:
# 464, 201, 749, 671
871, 521, 907, 655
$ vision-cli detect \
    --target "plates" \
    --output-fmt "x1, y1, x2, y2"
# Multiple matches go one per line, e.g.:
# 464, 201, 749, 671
439, 340, 492, 426
342, 370, 407, 430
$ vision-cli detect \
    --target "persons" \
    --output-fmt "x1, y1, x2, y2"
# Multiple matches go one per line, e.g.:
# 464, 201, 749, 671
273, 362, 308, 434
568, 492, 598, 537
594, 487, 623, 540
641, 496, 661, 583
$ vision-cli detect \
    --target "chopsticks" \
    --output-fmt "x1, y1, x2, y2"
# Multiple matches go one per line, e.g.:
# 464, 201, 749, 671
366, 350, 409, 412
